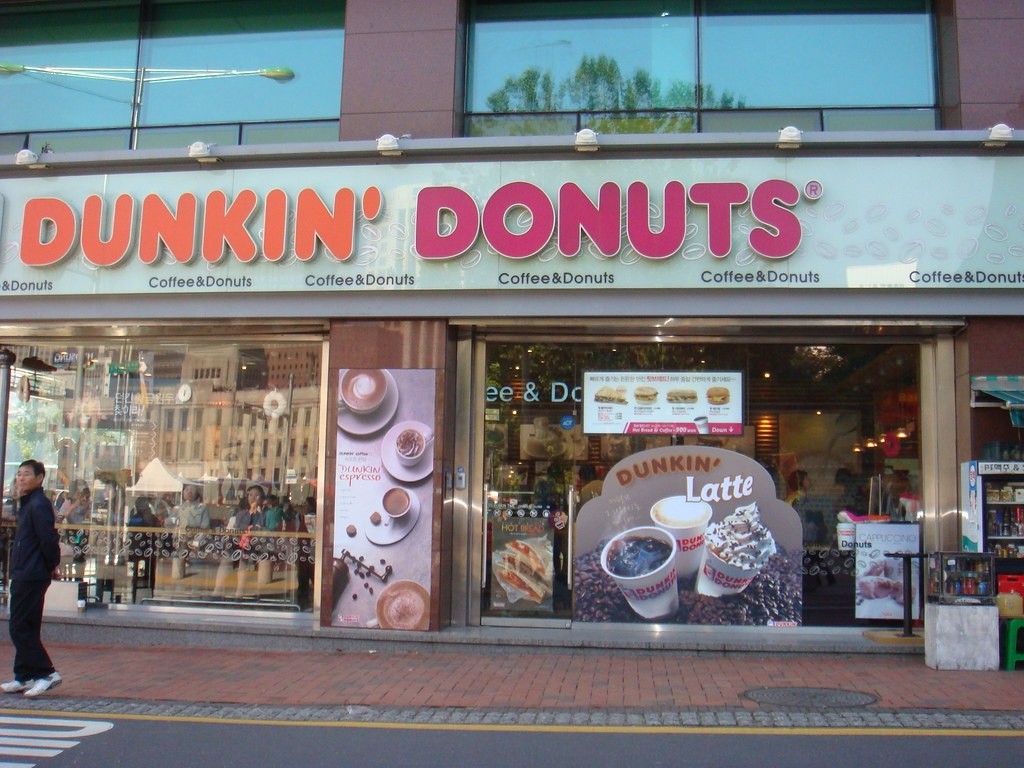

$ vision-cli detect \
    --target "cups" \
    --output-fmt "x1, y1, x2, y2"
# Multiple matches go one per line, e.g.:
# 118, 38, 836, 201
650, 496, 712, 579
534, 416, 548, 438
338, 369, 389, 414
601, 526, 679, 621
382, 486, 412, 526
366, 580, 429, 630
395, 428, 434, 466
837, 523, 855, 551
694, 541, 759, 596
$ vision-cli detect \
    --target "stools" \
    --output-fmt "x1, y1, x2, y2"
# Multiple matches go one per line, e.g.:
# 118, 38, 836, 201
1001, 618, 1024, 671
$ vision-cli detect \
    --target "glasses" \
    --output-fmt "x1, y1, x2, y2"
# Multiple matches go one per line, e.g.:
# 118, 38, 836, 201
15, 472, 33, 478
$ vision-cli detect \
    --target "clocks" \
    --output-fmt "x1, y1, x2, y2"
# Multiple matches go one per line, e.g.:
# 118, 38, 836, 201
178, 384, 192, 402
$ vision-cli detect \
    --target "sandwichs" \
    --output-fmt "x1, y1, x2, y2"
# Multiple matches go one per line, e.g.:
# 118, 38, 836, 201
595, 386, 629, 405
494, 538, 554, 603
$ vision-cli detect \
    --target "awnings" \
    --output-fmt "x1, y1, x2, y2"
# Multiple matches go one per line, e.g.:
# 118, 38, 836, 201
972, 377, 1024, 428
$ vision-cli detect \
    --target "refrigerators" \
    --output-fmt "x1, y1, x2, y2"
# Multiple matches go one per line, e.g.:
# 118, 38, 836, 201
961, 459, 1024, 558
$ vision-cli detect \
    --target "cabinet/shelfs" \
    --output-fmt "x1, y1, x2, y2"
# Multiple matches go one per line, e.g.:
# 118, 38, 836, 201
929, 460, 1024, 607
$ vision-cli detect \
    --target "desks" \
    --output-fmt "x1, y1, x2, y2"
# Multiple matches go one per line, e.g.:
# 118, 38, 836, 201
883, 552, 930, 636
0, 520, 316, 612
803, 543, 855, 586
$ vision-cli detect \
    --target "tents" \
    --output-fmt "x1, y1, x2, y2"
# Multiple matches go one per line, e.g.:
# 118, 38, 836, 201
127, 457, 241, 502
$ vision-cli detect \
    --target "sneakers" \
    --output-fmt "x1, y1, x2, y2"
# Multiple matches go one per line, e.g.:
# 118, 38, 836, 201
24, 671, 62, 697
0, 678, 35, 693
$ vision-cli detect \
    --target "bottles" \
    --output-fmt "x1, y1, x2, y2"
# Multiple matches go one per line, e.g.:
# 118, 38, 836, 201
929, 557, 990, 596
986, 481, 1024, 558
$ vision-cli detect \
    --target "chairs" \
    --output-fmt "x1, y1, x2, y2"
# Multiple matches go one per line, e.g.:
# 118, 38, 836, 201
997, 574, 1024, 597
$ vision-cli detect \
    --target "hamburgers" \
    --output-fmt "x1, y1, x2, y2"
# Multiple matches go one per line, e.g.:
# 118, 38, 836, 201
634, 387, 658, 404
707, 387, 730, 405
666, 389, 698, 403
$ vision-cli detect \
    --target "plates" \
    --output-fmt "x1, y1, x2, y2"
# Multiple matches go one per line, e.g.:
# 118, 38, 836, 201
365, 488, 420, 545
338, 369, 399, 435
381, 421, 434, 482
524, 441, 566, 458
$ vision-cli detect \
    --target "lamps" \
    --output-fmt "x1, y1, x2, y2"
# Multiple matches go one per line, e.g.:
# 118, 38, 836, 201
851, 387, 864, 452
876, 368, 886, 442
864, 378, 877, 447
895, 360, 910, 438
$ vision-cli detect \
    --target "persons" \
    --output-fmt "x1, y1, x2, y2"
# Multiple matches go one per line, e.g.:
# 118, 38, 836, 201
785, 470, 812, 505
835, 467, 866, 514
0, 459, 63, 698
213, 485, 314, 598
129, 498, 163, 600
58, 472, 121, 580
171, 482, 209, 529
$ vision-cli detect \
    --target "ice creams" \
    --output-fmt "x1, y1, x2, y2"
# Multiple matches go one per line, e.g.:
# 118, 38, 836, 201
694, 500, 776, 597
